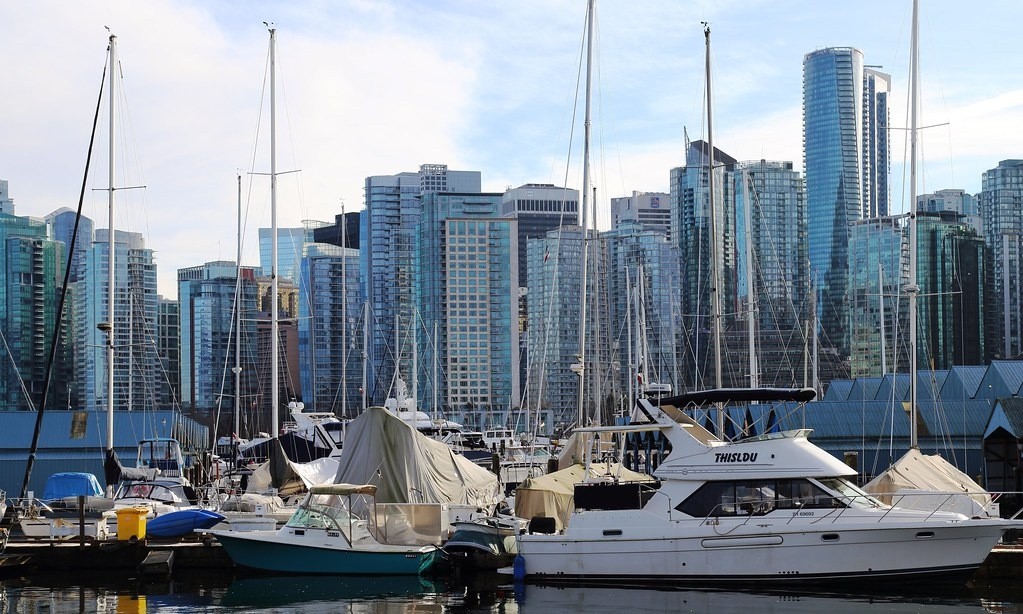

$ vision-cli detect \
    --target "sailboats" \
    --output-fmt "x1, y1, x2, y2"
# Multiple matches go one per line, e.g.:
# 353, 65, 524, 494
1, 1, 1021, 613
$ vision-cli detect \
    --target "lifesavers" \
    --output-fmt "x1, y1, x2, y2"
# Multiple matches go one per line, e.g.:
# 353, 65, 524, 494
133, 485, 148, 497
